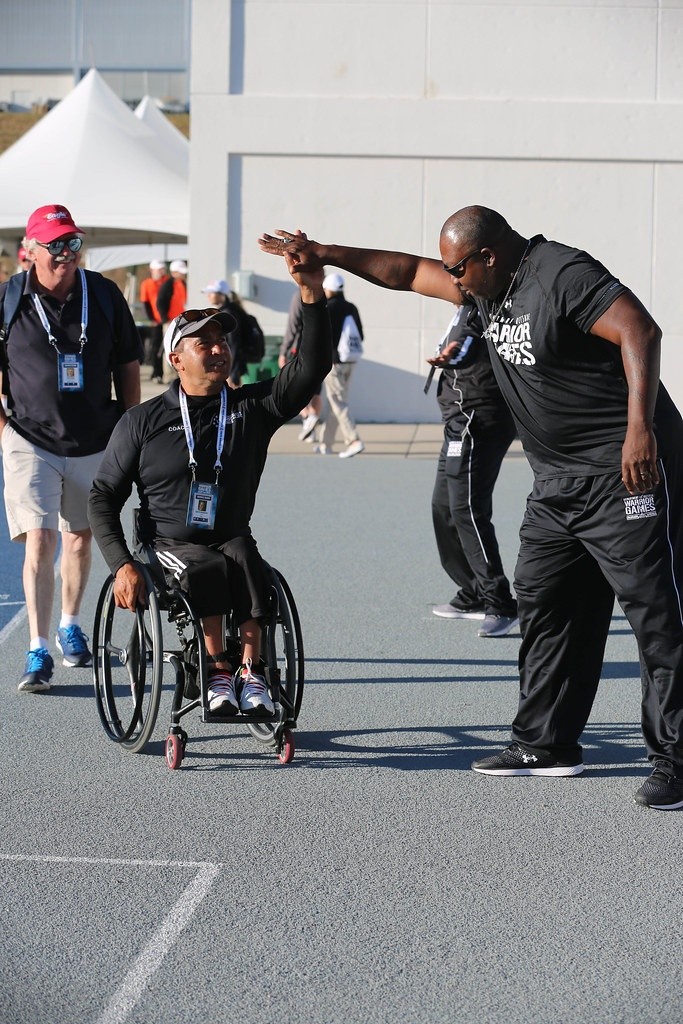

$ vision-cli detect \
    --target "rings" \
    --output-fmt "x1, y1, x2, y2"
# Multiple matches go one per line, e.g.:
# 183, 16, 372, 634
283, 237, 290, 244
641, 472, 647, 474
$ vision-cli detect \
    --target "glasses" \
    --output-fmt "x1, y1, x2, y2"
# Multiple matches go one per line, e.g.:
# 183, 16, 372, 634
170, 308, 221, 353
442, 242, 497, 279
35, 236, 83, 255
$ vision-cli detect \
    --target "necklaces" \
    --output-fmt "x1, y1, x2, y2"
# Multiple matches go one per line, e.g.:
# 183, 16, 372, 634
481, 239, 531, 340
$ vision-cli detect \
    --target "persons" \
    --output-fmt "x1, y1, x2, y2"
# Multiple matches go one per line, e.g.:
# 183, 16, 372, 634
279, 272, 364, 458
84, 229, 334, 719
140, 260, 171, 381
16, 247, 34, 272
200, 279, 249, 388
158, 258, 188, 383
258, 206, 683, 812
427, 304, 521, 635
67, 368, 74, 378
0, 205, 141, 692
199, 502, 206, 511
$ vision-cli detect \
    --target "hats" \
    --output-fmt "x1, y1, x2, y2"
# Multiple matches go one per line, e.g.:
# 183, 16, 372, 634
19, 247, 27, 259
201, 280, 231, 296
322, 273, 344, 292
163, 312, 238, 368
169, 260, 189, 275
25, 204, 86, 243
150, 259, 167, 269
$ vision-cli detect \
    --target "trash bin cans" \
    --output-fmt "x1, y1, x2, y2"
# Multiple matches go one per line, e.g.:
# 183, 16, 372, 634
245, 336, 282, 384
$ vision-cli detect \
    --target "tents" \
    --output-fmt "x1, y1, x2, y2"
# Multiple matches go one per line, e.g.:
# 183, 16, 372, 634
0, 65, 191, 314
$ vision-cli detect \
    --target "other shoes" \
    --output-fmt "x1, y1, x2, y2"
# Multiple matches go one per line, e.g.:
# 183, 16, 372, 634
313, 444, 333, 455
153, 376, 162, 384
339, 441, 365, 459
300, 414, 322, 442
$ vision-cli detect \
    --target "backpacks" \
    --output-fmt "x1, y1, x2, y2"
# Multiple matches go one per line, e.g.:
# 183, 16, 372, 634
244, 315, 265, 363
336, 315, 364, 362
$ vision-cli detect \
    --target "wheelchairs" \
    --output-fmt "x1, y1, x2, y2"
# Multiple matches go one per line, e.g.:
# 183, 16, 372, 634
89, 505, 305, 770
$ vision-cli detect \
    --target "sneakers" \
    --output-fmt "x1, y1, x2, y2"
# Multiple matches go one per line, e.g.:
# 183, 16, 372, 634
471, 742, 583, 776
236, 658, 276, 717
206, 670, 239, 715
18, 647, 54, 692
432, 602, 486, 620
478, 614, 519, 637
634, 765, 683, 810
55, 623, 93, 667
